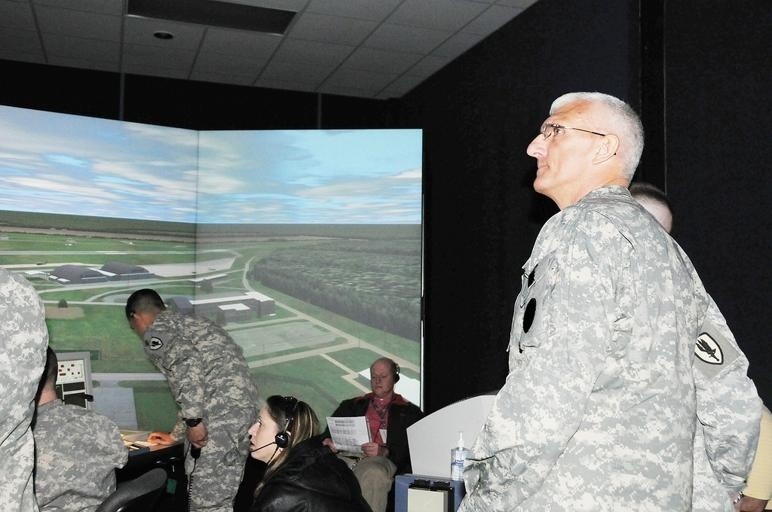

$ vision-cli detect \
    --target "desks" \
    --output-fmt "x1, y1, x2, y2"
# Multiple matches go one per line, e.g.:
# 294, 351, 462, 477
115, 429, 188, 511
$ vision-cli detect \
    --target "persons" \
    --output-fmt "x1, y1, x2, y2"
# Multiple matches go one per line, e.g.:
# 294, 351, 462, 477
34, 347, 128, 512
125, 289, 257, 512
0, 262, 52, 511
626, 180, 771, 511
246, 394, 373, 512
453, 92, 768, 512
323, 357, 425, 511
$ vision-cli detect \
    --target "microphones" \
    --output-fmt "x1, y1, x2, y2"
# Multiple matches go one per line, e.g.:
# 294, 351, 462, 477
248, 440, 277, 453
62, 393, 94, 401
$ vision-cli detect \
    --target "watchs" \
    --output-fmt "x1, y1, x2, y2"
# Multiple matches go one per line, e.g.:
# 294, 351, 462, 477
184, 418, 203, 427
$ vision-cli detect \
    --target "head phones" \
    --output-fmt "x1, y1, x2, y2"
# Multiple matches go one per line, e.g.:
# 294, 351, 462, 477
275, 397, 298, 448
394, 362, 399, 383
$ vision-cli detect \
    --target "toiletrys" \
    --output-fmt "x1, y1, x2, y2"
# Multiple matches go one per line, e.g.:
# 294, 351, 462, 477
451, 429, 471, 482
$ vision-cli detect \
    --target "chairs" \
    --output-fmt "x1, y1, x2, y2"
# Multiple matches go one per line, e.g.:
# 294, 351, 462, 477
89, 465, 172, 512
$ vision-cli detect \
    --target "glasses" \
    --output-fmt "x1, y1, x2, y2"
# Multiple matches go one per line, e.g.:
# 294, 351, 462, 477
540, 122, 608, 138
